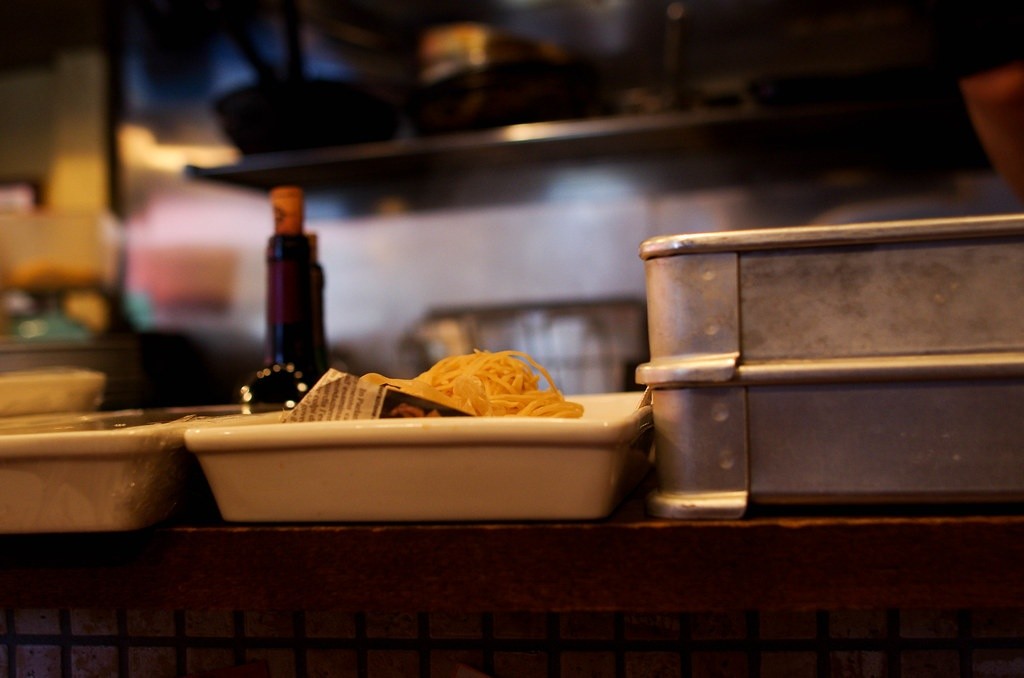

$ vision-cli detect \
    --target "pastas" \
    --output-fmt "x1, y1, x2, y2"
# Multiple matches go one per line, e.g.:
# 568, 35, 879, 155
413, 346, 584, 420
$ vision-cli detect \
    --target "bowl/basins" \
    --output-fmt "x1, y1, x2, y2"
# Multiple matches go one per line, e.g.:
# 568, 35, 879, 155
0, 374, 106, 417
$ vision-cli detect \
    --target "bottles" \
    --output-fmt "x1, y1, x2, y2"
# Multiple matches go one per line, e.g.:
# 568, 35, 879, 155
305, 232, 326, 393
266, 187, 318, 382
